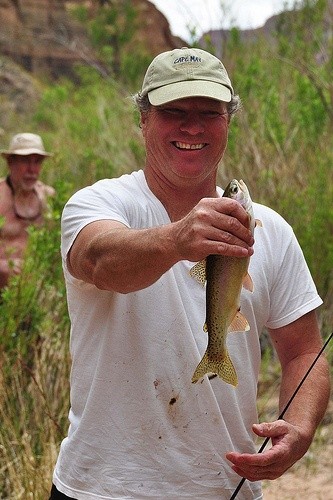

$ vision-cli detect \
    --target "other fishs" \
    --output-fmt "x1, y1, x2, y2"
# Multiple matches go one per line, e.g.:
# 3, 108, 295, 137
188, 177, 263, 388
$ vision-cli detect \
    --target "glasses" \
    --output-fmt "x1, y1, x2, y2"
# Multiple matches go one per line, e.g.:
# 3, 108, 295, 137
6, 173, 43, 221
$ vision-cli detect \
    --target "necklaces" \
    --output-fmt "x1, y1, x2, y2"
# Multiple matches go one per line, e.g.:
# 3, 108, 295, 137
6, 173, 42, 223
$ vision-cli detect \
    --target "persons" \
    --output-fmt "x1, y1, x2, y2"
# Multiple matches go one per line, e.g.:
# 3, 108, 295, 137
0, 132, 57, 387
48, 47, 331, 500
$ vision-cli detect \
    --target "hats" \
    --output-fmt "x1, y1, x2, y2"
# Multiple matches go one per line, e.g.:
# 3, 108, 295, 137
0, 132, 53, 159
139, 45, 235, 107
